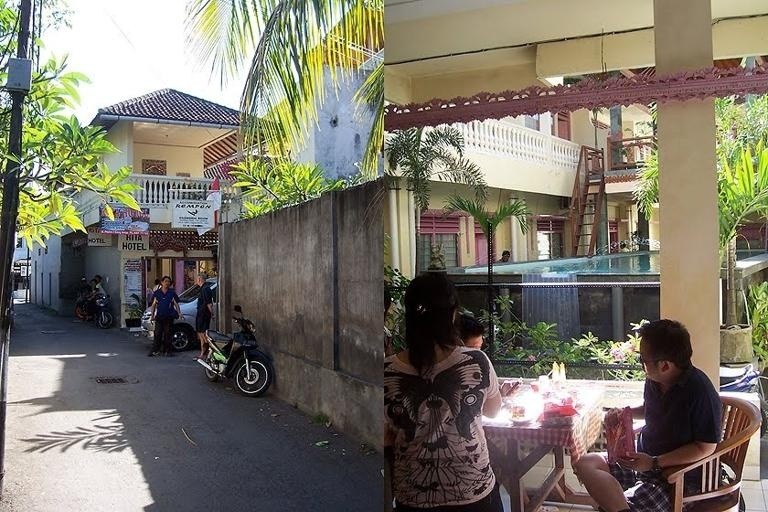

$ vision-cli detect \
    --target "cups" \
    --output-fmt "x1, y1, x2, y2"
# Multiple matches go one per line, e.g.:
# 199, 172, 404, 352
512, 399, 526, 417
538, 374, 550, 397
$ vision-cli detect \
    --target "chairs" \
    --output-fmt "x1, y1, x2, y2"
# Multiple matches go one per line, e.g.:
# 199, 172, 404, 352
634, 395, 762, 512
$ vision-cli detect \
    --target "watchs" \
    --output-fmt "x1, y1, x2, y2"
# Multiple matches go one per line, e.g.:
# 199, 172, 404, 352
651, 454, 663, 475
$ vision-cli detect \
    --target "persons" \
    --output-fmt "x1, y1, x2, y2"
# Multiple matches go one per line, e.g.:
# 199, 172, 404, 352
499, 250, 512, 263
454, 312, 486, 352
193, 271, 214, 359
384, 269, 504, 510
153, 279, 162, 292
574, 318, 724, 511
150, 275, 184, 358
78, 274, 107, 326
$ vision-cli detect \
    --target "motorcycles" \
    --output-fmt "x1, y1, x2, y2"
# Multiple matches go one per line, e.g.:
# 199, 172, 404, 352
74, 287, 114, 328
197, 304, 274, 398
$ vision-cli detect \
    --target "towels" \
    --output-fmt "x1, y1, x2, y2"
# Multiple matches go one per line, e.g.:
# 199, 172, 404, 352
604, 407, 634, 464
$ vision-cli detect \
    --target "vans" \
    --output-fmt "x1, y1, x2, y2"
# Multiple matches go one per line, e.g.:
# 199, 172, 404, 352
140, 278, 217, 349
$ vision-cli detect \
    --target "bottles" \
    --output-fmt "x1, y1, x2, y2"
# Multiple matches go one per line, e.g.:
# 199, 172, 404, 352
551, 361, 566, 382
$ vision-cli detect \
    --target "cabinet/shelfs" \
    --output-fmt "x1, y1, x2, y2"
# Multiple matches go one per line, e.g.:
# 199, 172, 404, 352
480, 385, 603, 512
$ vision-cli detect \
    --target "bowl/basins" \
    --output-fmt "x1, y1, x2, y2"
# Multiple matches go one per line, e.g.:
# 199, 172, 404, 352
529, 382, 537, 392
